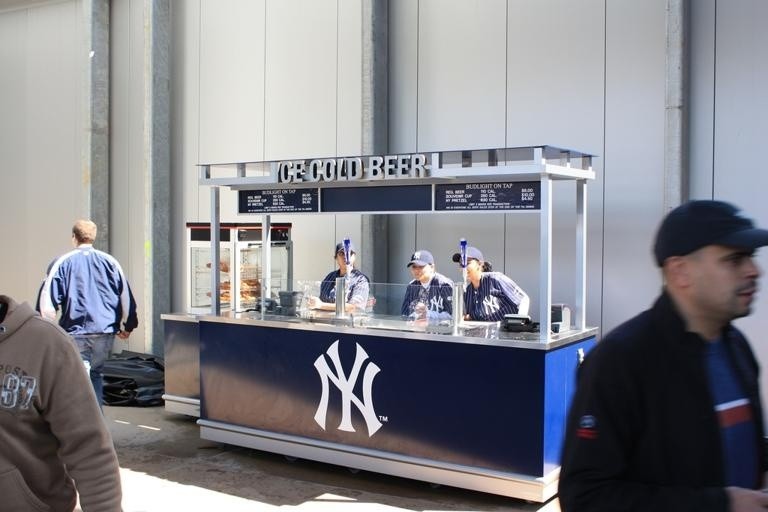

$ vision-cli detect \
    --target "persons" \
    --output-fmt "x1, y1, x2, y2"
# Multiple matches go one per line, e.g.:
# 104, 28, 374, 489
398, 250, 459, 323
0, 279, 123, 511
452, 246, 530, 324
306, 241, 370, 315
558, 199, 767, 510
35, 221, 138, 415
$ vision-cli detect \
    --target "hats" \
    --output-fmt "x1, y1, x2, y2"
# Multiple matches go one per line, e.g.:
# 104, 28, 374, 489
336, 242, 356, 252
654, 200, 768, 269
407, 250, 434, 268
453, 247, 484, 262
